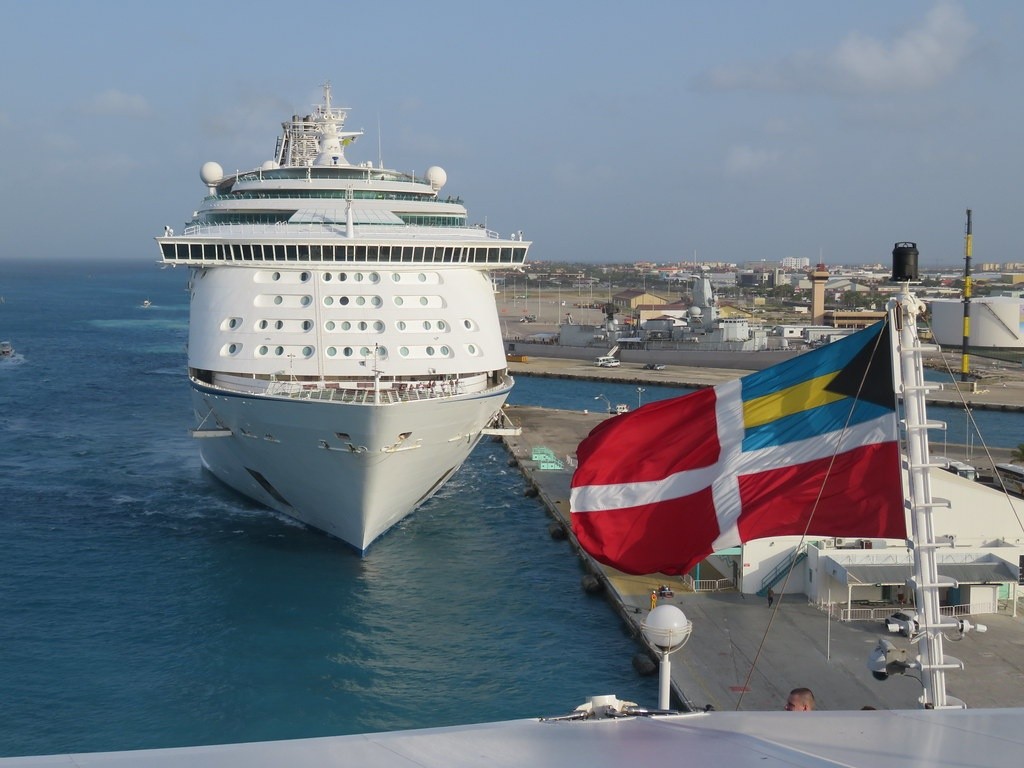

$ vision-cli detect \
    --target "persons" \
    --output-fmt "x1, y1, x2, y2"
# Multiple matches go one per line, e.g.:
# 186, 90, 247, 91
897, 585, 905, 604
783, 688, 817, 712
492, 412, 505, 429
767, 586, 774, 608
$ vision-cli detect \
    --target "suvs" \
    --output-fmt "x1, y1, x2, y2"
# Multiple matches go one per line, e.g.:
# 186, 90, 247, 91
604, 360, 620, 368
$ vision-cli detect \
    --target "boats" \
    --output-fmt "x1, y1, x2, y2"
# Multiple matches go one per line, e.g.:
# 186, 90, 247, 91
0, 342, 15, 358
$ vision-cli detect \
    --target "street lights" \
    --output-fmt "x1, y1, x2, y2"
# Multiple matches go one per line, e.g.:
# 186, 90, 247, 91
594, 394, 611, 419
635, 387, 645, 409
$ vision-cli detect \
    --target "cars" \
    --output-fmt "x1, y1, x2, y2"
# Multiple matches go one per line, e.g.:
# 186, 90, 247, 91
642, 363, 666, 370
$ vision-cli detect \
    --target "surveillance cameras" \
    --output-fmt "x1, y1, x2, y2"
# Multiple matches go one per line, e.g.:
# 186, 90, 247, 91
868, 648, 889, 680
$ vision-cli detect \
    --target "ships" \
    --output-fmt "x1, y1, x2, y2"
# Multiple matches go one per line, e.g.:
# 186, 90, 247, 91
151, 79, 535, 561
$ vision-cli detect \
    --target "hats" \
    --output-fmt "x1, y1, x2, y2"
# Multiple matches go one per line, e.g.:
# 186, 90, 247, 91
652, 591, 655, 593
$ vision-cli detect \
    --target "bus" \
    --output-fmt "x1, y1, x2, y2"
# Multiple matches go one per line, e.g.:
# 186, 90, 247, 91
594, 356, 614, 367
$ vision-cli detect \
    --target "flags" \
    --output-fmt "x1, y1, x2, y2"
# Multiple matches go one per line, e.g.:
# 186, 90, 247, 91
567, 309, 906, 578
